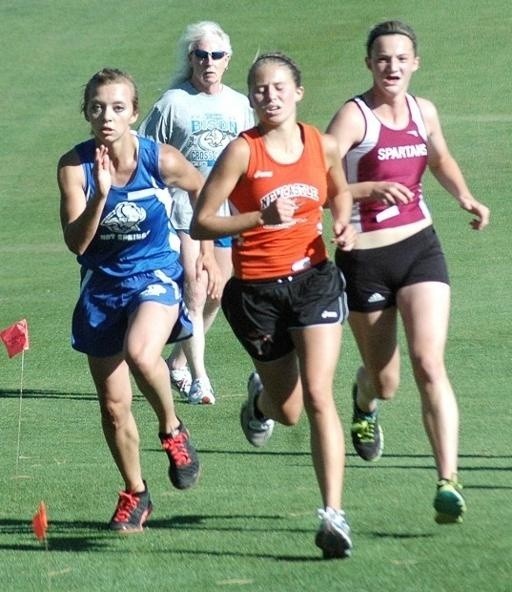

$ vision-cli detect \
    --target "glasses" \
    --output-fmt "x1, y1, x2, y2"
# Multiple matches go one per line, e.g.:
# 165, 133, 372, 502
190, 48, 229, 60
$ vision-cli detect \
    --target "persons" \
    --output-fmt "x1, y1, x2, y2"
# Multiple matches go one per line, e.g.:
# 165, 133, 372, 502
322, 22, 490, 524
189, 51, 354, 556
55, 69, 221, 535
136, 21, 256, 403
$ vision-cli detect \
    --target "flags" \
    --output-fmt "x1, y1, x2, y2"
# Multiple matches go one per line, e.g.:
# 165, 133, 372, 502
1, 319, 30, 359
31, 500, 49, 543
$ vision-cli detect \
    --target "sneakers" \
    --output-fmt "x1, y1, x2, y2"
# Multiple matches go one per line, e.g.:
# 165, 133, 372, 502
238, 370, 276, 449
164, 357, 217, 407
157, 414, 202, 491
313, 505, 354, 562
349, 380, 386, 464
431, 471, 467, 526
105, 478, 155, 534
